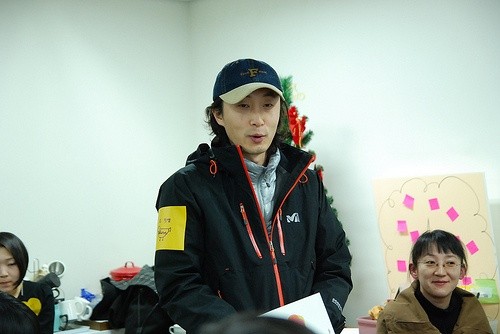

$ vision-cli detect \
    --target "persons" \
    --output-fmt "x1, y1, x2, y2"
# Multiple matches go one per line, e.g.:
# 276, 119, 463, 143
0, 232, 55, 334
377, 230, 495, 334
155, 59, 354, 334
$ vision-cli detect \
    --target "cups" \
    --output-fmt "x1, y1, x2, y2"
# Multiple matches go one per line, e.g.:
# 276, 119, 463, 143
53, 302, 68, 332
169, 324, 186, 334
76, 298, 92, 322
60, 300, 83, 321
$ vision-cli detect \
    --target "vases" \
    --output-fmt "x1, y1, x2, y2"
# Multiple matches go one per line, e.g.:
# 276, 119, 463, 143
356, 316, 377, 334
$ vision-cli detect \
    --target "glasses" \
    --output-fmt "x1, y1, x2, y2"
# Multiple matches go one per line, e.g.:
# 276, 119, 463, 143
416, 256, 462, 269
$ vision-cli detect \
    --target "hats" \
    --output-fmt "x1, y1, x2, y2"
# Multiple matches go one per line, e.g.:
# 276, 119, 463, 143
213, 59, 286, 104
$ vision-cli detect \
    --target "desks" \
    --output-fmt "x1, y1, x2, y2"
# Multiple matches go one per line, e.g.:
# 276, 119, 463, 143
55, 318, 125, 334
